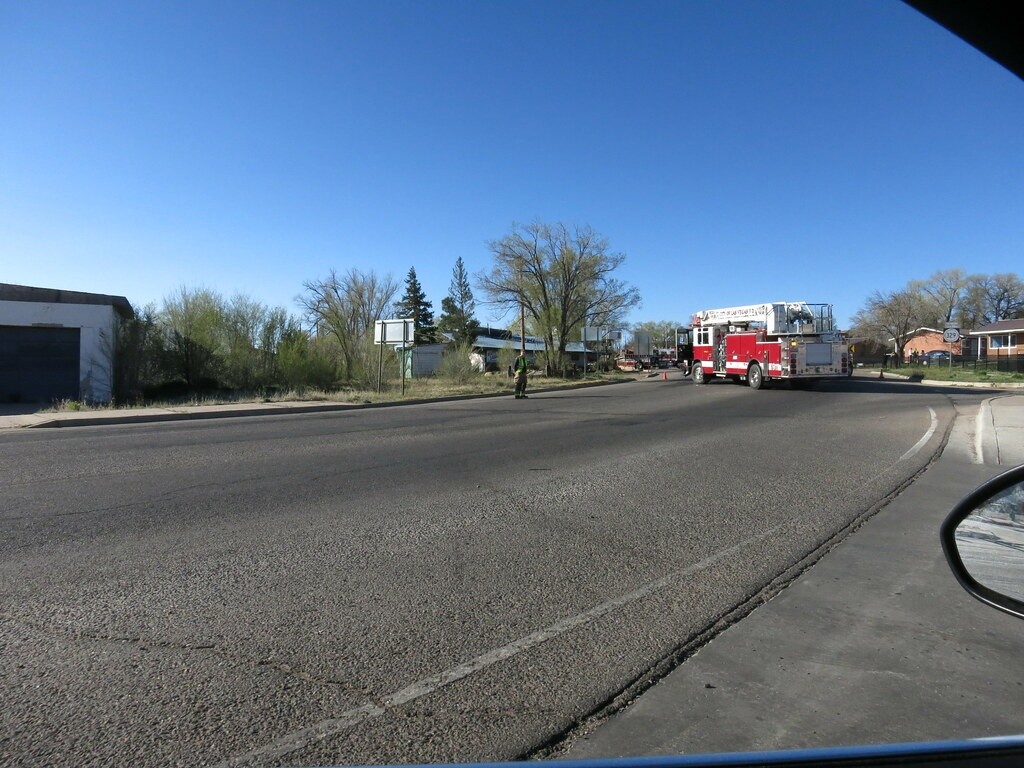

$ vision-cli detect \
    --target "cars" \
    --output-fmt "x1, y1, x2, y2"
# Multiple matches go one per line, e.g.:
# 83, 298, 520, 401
917, 349, 968, 366
857, 362, 864, 368
618, 356, 674, 369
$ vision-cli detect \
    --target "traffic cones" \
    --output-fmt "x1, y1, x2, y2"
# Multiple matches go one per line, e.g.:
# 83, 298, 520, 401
663, 371, 667, 380
878, 369, 884, 379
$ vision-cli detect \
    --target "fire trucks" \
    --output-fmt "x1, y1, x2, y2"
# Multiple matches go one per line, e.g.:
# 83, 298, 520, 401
676, 302, 856, 390
658, 349, 676, 366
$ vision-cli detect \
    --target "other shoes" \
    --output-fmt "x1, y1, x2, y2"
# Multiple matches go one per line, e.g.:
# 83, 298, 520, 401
521, 395, 528, 398
516, 395, 522, 399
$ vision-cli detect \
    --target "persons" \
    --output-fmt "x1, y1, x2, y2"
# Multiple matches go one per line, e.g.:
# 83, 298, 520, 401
909, 348, 926, 363
514, 349, 528, 399
